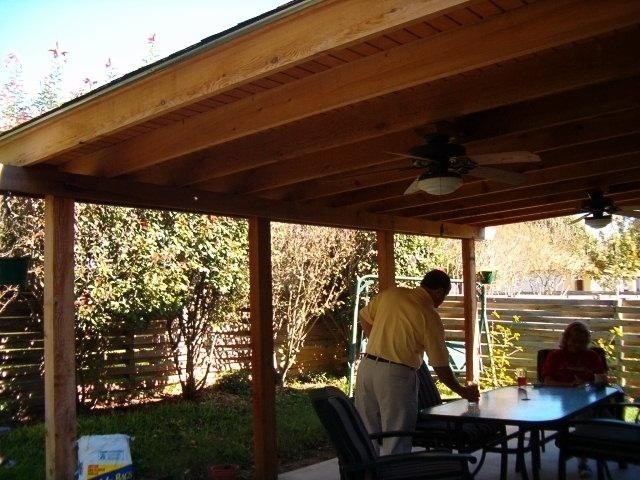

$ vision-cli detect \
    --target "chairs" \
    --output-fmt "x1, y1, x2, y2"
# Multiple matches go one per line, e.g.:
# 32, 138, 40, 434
413, 361, 508, 480
554, 401, 640, 480
309, 387, 477, 480
531, 347, 610, 454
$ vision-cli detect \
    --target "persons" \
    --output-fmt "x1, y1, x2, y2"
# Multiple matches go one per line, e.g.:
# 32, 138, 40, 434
540, 319, 610, 479
354, 270, 480, 455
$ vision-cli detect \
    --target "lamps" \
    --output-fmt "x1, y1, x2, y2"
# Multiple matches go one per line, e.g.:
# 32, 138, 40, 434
585, 217, 612, 228
417, 170, 464, 196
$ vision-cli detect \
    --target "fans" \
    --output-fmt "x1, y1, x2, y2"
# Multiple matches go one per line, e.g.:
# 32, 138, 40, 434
326, 128, 541, 196
566, 178, 640, 223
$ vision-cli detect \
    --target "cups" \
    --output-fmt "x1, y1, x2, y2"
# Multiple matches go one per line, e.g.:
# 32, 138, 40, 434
467, 380, 479, 404
517, 367, 527, 389
594, 373, 604, 385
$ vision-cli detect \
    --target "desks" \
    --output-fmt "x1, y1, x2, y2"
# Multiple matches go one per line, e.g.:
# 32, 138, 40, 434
419, 384, 618, 480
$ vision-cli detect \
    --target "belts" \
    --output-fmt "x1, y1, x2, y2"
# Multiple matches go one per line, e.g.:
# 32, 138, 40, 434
363, 352, 403, 365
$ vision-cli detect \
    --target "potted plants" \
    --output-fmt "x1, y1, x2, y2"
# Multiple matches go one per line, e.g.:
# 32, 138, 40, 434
208, 455, 241, 480
